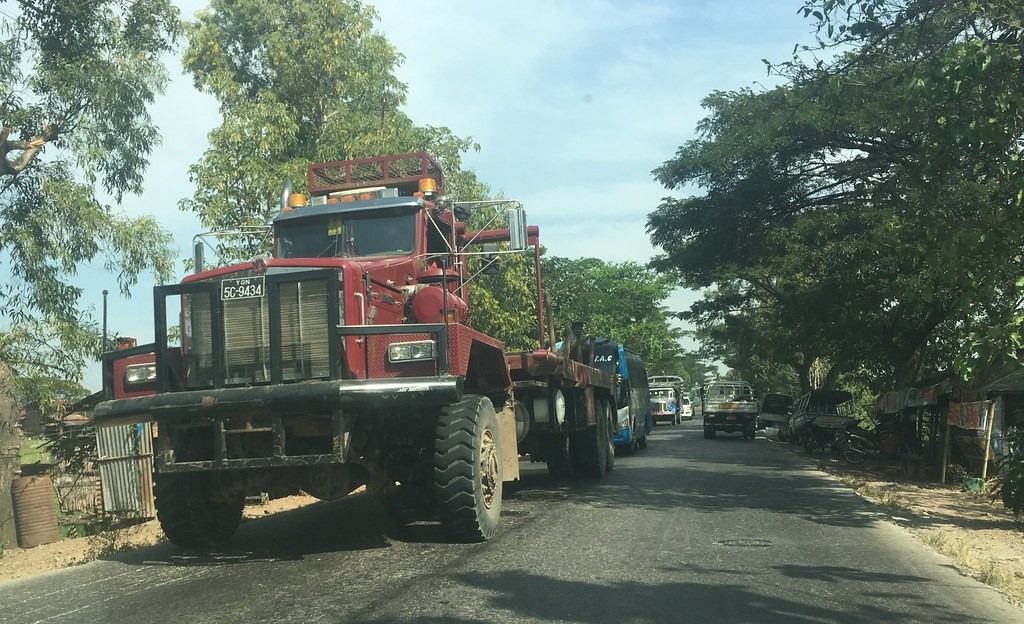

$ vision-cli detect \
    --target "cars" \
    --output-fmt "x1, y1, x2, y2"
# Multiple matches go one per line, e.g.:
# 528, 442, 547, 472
682, 396, 696, 421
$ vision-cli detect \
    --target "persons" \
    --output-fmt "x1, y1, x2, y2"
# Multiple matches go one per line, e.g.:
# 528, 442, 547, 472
560, 320, 596, 365
387, 224, 439, 271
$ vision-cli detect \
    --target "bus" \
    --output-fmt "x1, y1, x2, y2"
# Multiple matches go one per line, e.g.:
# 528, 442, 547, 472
546, 336, 652, 456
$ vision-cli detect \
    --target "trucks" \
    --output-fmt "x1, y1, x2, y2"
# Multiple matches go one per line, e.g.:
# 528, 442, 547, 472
699, 380, 760, 440
647, 375, 685, 426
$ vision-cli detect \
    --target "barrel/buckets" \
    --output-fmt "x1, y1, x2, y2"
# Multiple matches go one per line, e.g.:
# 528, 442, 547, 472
12, 475, 60, 548
903, 456, 928, 481
962, 476, 981, 489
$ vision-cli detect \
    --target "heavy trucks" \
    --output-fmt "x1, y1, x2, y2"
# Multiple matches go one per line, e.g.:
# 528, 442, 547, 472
86, 150, 622, 552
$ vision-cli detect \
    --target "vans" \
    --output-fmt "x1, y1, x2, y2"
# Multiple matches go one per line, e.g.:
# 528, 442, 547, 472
755, 391, 795, 439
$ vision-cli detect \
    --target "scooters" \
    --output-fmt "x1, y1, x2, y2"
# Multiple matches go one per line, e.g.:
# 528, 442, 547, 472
837, 413, 913, 466
